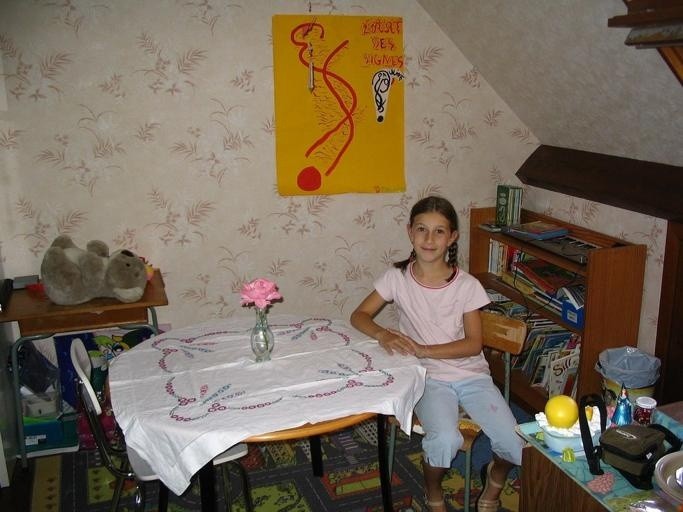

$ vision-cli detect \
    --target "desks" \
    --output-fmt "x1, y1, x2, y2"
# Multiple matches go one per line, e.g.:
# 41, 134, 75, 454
104, 316, 418, 512
1, 269, 168, 472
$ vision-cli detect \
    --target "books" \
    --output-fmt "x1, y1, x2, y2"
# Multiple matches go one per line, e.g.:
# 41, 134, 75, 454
475, 180, 602, 407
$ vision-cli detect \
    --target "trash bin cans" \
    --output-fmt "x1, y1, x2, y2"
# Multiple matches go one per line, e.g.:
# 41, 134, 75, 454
599, 347, 661, 407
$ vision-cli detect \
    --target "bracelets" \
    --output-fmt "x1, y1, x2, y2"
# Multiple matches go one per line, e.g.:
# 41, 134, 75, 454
423, 345, 429, 360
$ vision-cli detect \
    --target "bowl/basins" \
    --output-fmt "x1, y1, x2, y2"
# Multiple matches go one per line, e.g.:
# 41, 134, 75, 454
653, 450, 683, 504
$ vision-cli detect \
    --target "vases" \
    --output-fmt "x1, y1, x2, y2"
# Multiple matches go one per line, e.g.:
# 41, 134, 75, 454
250, 307, 275, 363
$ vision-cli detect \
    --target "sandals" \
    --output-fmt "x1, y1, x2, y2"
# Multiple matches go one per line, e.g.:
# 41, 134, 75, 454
475, 460, 499, 512
423, 492, 445, 512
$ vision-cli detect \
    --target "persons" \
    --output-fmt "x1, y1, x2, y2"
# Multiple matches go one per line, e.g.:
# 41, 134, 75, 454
349, 195, 526, 512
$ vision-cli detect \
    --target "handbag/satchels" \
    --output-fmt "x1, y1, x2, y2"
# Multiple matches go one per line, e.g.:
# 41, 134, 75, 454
599, 424, 682, 489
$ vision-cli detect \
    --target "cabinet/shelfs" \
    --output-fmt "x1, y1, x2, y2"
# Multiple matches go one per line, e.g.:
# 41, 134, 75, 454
470, 226, 647, 417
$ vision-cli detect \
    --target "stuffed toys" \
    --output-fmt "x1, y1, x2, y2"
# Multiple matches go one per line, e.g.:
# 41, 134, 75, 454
37, 231, 148, 306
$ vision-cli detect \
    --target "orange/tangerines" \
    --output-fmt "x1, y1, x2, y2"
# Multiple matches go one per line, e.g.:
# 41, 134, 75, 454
544, 395, 580, 429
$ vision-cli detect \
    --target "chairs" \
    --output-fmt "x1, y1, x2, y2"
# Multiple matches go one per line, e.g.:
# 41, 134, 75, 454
69, 337, 251, 512
388, 310, 527, 511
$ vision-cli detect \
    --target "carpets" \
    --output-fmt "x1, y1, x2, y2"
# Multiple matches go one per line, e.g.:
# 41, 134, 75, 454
22, 392, 536, 510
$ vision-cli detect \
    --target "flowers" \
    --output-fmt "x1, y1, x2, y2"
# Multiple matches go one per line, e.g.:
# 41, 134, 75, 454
239, 277, 283, 351
